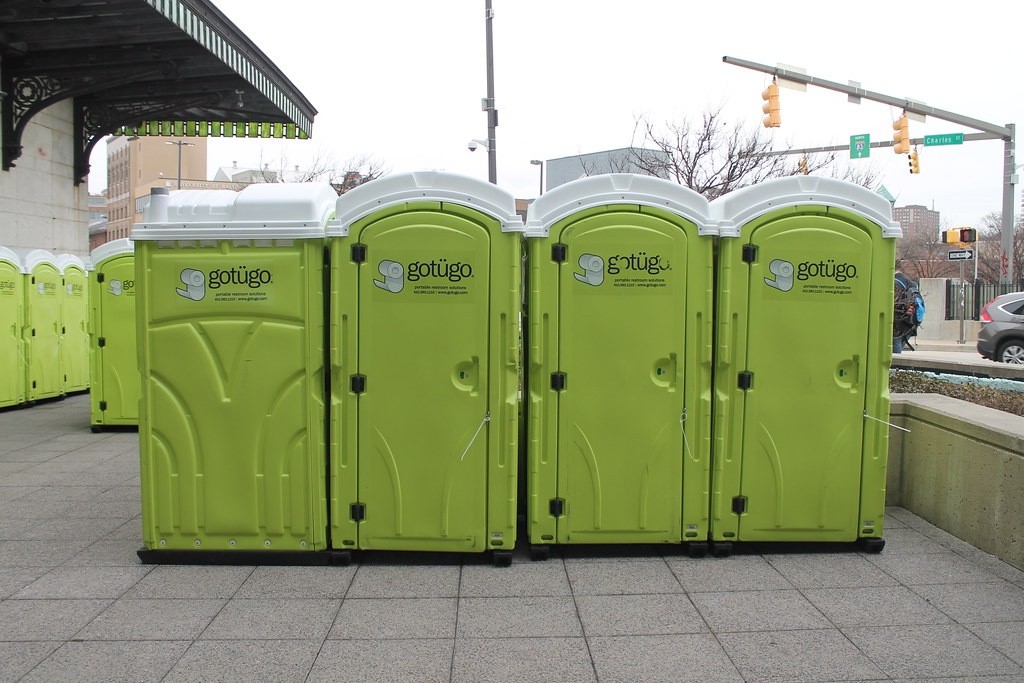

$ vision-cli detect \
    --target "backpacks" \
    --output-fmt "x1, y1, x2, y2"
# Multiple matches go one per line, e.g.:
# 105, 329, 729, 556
895, 277, 925, 326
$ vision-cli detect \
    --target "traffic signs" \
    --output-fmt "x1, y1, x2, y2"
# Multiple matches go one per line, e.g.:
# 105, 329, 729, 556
923, 132, 963, 146
947, 249, 974, 261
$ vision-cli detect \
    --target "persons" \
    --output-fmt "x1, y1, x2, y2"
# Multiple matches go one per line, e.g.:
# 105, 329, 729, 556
893, 258, 917, 354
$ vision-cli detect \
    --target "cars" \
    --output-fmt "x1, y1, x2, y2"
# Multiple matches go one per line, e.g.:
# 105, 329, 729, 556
978, 292, 1024, 363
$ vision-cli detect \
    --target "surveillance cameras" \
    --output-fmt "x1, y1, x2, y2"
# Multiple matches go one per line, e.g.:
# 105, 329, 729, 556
468, 142, 477, 152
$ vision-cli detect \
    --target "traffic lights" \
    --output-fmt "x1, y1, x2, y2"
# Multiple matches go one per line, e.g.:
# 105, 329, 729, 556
908, 152, 920, 174
960, 229, 977, 242
893, 115, 910, 154
762, 84, 781, 128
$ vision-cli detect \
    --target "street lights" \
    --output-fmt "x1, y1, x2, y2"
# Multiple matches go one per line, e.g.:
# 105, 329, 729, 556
165, 140, 195, 190
530, 160, 543, 196
